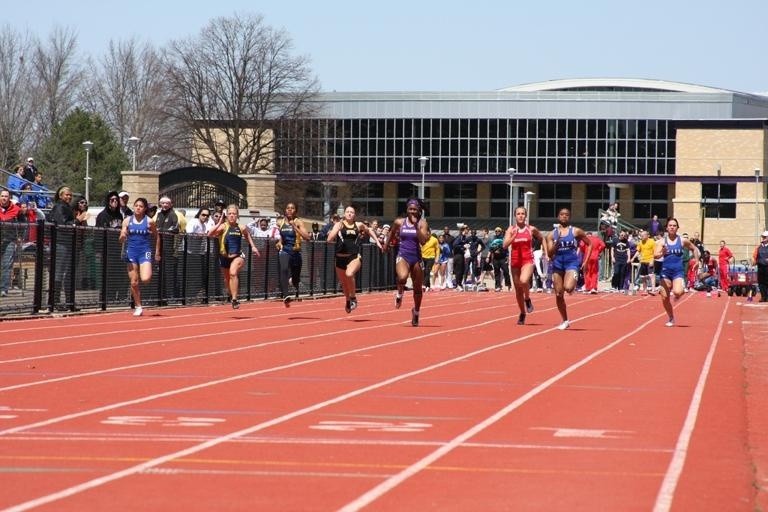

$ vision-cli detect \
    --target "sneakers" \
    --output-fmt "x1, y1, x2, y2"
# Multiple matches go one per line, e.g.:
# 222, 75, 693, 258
665, 319, 675, 326
349, 297, 357, 310
517, 313, 526, 325
395, 296, 402, 309
345, 301, 351, 313
525, 298, 533, 313
231, 300, 240, 309
283, 295, 292, 308
0, 290, 8, 297
133, 306, 142, 316
412, 308, 418, 326
557, 319, 569, 330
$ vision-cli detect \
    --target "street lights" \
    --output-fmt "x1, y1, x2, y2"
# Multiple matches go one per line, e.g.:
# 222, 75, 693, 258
508, 168, 516, 229
525, 191, 535, 229
81, 140, 95, 205
753, 167, 761, 246
417, 156, 430, 200
129, 137, 141, 170
151, 154, 160, 171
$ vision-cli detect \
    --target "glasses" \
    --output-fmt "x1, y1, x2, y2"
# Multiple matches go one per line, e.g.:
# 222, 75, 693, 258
150, 208, 156, 212
214, 214, 221, 218
110, 198, 118, 202
202, 214, 209, 217
77, 201, 88, 204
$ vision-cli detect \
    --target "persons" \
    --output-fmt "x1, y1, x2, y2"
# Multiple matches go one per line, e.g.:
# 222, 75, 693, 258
178, 207, 210, 303
326, 205, 384, 313
683, 229, 699, 293
699, 240, 718, 296
207, 205, 260, 309
430, 224, 494, 292
502, 206, 553, 325
116, 192, 130, 304
310, 222, 324, 240
654, 216, 663, 296
383, 222, 399, 252
533, 239, 553, 293
47, 185, 91, 313
177, 208, 189, 303
361, 216, 382, 255
70, 196, 88, 290
318, 213, 340, 241
420, 229, 430, 292
31, 172, 53, 208
752, 230, 768, 303
12, 202, 29, 287
208, 211, 227, 305
25, 199, 46, 244
150, 197, 186, 306
382, 198, 430, 326
19, 183, 45, 208
7, 164, 26, 201
654, 216, 699, 327
546, 206, 592, 330
269, 214, 284, 294
145, 202, 158, 219
576, 232, 592, 294
494, 227, 511, 292
215, 200, 226, 215
718, 240, 734, 291
93, 191, 123, 307
0, 189, 26, 297
275, 203, 311, 308
119, 198, 161, 317
592, 203, 654, 295
247, 217, 271, 296
21, 158, 38, 182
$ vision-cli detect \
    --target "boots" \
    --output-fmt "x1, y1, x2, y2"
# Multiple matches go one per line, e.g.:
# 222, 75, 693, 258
47, 281, 68, 312
66, 282, 80, 312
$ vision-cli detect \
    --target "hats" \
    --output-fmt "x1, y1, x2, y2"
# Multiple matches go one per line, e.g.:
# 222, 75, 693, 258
215, 199, 226, 208
312, 222, 318, 227
118, 191, 129, 198
28, 157, 33, 162
148, 203, 157, 210
761, 231, 768, 236
